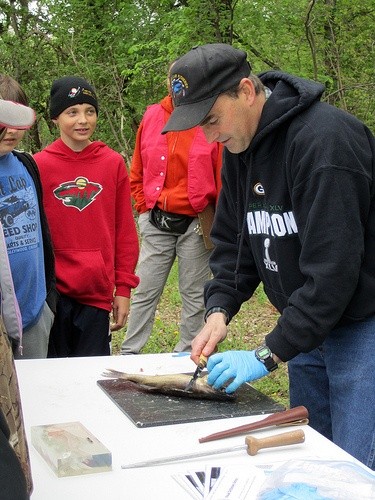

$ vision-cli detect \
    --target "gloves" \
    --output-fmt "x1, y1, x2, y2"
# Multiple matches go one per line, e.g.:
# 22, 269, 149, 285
207, 350, 270, 394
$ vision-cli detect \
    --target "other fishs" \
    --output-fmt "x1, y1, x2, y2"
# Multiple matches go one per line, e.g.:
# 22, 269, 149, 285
102, 367, 239, 401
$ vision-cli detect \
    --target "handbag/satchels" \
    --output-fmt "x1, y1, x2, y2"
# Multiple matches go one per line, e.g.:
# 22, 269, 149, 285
148, 205, 195, 235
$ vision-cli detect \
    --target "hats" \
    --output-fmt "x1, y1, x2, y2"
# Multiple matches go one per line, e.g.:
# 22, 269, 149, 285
160, 44, 252, 134
0, 99, 36, 130
50, 77, 99, 121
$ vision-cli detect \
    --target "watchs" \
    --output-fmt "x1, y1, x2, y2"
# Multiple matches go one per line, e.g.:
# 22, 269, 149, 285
255, 345, 278, 372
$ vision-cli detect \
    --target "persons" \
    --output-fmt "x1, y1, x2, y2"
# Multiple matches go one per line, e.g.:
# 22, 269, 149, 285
121, 61, 223, 354
32, 76, 139, 358
161, 43, 375, 471
0, 75, 57, 359
0, 100, 36, 500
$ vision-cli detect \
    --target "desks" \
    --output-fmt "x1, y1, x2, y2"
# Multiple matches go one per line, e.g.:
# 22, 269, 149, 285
12, 351, 375, 500
